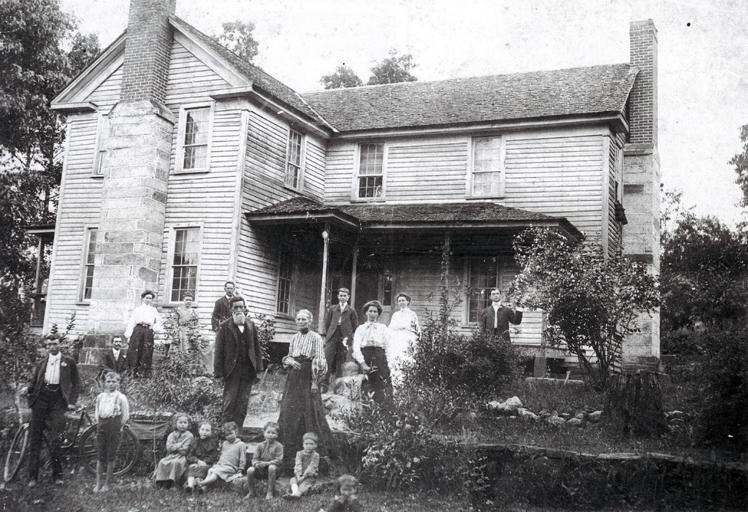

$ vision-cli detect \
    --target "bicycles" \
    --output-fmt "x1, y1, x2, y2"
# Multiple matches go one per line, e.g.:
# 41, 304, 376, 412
3, 387, 144, 482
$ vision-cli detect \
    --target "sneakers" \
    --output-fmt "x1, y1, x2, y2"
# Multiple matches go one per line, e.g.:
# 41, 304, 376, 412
27, 479, 38, 489
54, 478, 65, 487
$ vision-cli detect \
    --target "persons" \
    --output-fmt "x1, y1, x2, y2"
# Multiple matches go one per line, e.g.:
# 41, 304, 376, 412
478, 288, 524, 343
319, 287, 359, 393
95, 335, 130, 388
92, 370, 131, 493
351, 300, 393, 411
26, 335, 81, 486
277, 308, 338, 456
123, 289, 161, 378
388, 294, 422, 383
168, 291, 201, 364
153, 411, 322, 500
327, 472, 365, 511
211, 281, 248, 330
213, 295, 264, 427
